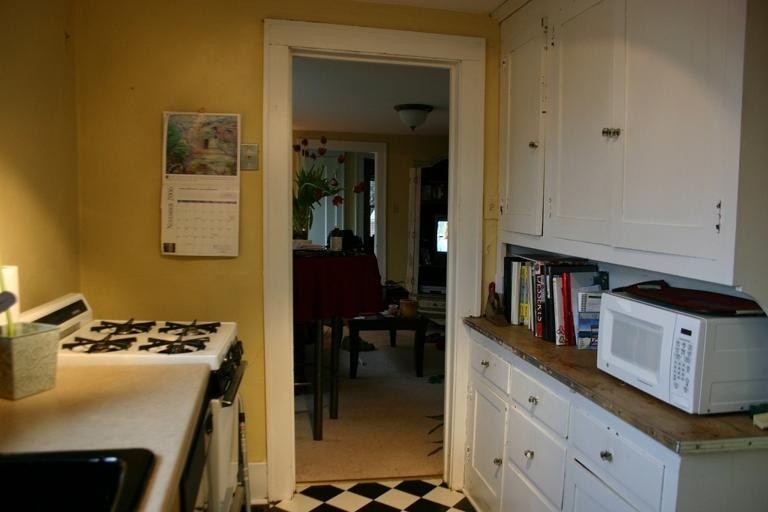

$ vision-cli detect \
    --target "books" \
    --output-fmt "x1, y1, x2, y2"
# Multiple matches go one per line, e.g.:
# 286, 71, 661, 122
500, 255, 608, 349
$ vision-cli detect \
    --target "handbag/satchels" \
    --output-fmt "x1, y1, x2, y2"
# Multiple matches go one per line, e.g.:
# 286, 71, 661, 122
382, 280, 409, 310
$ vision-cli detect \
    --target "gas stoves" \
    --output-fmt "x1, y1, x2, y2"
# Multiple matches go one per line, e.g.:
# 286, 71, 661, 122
57, 319, 247, 405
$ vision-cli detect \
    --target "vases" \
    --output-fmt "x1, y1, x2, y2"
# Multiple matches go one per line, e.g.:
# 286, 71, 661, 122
293, 214, 308, 238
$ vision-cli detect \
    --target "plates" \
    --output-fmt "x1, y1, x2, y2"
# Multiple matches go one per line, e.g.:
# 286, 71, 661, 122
0, 447, 156, 512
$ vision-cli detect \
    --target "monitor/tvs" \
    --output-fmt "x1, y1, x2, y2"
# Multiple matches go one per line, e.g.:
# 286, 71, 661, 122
434, 215, 448, 255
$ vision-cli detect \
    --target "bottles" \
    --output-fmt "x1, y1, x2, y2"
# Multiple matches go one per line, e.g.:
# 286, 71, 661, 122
329, 226, 344, 252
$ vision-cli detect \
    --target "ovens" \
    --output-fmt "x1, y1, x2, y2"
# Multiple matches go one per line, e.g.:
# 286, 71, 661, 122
210, 359, 247, 511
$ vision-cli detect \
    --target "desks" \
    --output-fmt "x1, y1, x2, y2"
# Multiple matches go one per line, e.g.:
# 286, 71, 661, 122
348, 308, 426, 380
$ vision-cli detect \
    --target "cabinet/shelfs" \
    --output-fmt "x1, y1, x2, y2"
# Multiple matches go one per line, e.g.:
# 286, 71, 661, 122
546, 3, 764, 296
464, 323, 679, 512
501, 7, 544, 247
293, 246, 418, 442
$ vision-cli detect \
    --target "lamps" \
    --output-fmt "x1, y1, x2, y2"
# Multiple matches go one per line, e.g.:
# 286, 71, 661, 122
393, 103, 433, 133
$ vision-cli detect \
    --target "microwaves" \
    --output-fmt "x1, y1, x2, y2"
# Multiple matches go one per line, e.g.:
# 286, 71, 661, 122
596, 280, 767, 416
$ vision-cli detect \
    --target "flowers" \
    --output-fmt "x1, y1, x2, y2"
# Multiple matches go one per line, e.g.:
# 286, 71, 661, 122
292, 136, 369, 218
